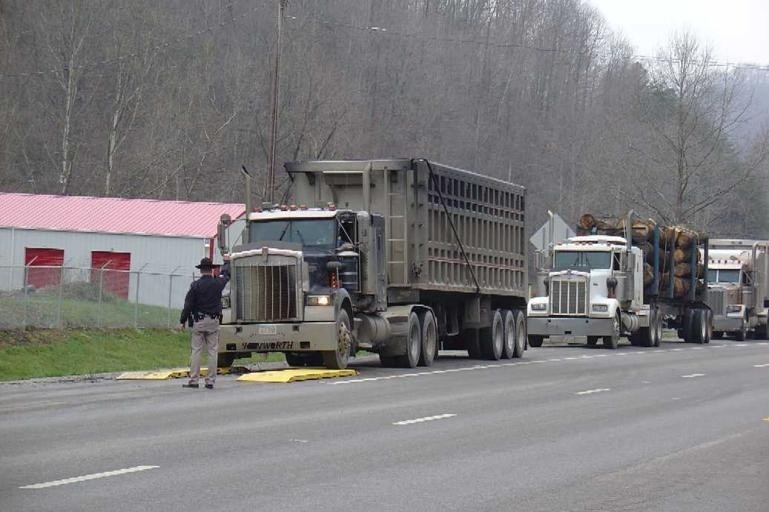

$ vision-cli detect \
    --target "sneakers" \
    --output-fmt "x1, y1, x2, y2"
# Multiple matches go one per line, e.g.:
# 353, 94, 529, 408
205, 384, 213, 388
183, 384, 199, 388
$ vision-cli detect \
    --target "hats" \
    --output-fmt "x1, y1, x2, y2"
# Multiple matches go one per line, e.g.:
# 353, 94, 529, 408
195, 258, 218, 269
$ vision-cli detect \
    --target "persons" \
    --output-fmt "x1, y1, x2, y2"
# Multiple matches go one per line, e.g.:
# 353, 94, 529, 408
180, 254, 231, 388
